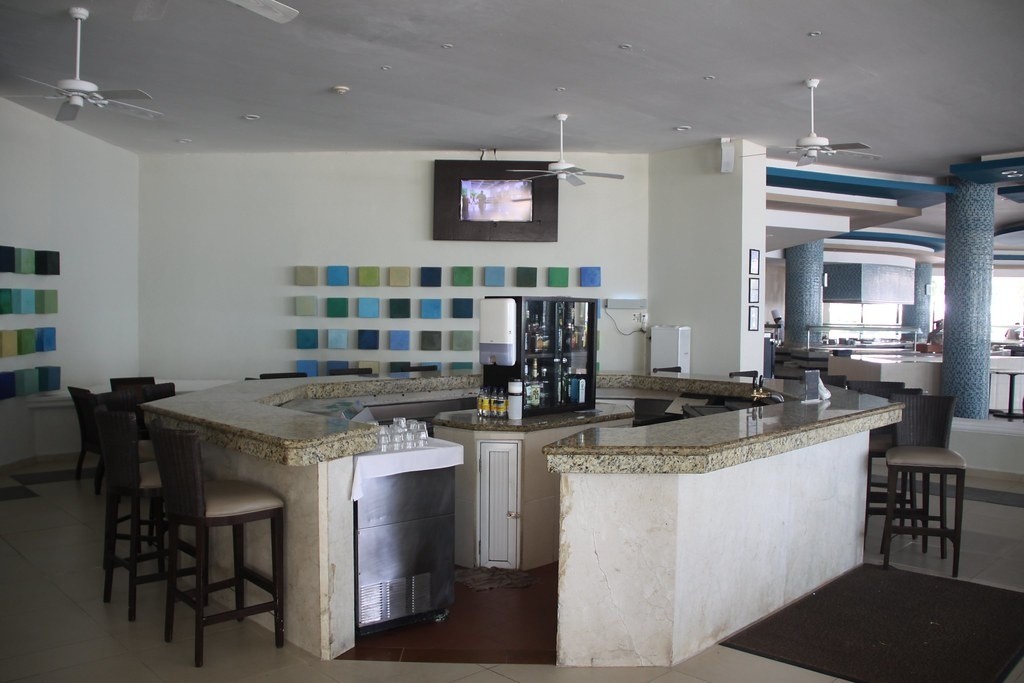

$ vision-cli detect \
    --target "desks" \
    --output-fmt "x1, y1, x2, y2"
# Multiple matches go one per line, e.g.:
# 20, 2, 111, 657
990, 370, 1024, 422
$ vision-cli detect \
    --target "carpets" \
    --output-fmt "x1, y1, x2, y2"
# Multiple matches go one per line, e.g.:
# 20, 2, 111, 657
716, 562, 1024, 683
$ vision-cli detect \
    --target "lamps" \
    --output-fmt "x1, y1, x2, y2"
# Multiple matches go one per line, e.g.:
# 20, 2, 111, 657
924, 283, 931, 296
821, 272, 828, 287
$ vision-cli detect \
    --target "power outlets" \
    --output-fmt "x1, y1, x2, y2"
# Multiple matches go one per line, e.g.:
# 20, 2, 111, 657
640, 312, 648, 323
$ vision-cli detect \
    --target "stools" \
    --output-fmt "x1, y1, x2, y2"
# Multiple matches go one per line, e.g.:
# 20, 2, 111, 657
94, 407, 209, 625
880, 395, 967, 577
859, 386, 924, 551
153, 425, 287, 667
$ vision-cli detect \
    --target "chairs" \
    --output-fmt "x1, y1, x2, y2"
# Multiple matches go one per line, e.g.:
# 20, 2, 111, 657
66, 377, 177, 495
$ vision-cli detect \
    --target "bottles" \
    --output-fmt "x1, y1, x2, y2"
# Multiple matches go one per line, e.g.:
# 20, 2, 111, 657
525, 310, 551, 353
558, 307, 587, 352
524, 357, 552, 410
477, 386, 507, 418
562, 358, 587, 404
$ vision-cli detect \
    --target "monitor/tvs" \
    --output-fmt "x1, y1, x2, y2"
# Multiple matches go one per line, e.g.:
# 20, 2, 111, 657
460, 180, 533, 222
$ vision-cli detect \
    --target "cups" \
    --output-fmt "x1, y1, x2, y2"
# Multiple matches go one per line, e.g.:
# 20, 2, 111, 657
508, 382, 522, 420
366, 417, 428, 452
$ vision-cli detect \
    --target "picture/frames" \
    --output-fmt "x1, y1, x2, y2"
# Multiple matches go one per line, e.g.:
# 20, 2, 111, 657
749, 277, 760, 303
748, 305, 759, 331
749, 249, 760, 275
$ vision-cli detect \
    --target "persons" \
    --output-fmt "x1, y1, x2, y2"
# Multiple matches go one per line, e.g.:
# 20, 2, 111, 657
927, 322, 944, 346
771, 309, 785, 345
476, 190, 488, 214
1005, 323, 1023, 341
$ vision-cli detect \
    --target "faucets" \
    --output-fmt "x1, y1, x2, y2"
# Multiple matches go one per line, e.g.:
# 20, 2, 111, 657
728, 371, 786, 403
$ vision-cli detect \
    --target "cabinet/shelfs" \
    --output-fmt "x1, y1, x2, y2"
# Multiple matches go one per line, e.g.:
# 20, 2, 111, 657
476, 438, 523, 568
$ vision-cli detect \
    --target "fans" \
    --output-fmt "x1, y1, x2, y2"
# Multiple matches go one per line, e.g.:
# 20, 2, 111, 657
738, 79, 883, 167
1, 7, 162, 122
504, 114, 624, 187
